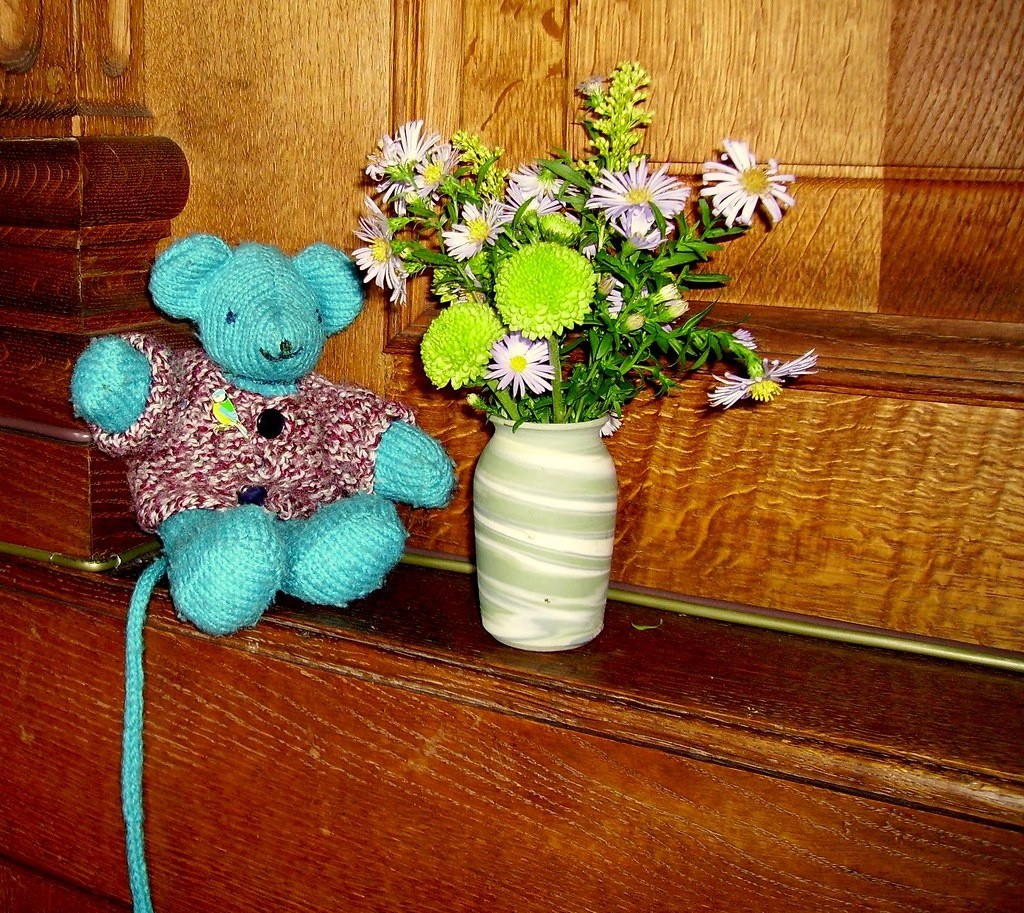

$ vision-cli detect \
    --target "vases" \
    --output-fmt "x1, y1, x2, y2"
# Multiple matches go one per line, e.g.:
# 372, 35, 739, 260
474, 406, 615, 658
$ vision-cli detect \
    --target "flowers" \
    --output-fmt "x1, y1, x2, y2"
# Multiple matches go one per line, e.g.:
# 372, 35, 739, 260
357, 61, 822, 441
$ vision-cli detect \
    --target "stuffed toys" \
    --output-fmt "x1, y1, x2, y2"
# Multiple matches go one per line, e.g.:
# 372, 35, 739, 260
72, 235, 454, 913
